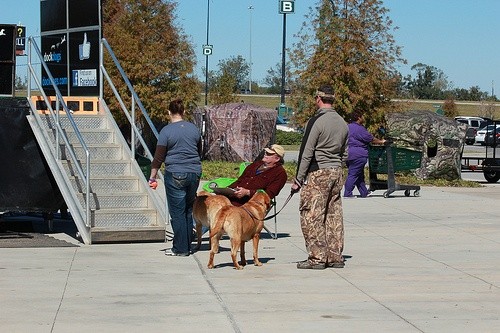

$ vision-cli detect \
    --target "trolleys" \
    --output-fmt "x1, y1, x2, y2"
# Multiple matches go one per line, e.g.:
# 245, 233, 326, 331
367, 141, 423, 198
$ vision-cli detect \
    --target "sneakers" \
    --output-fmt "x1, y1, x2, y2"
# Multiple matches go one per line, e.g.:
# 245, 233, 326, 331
296, 260, 326, 269
325, 262, 344, 268
164, 248, 193, 257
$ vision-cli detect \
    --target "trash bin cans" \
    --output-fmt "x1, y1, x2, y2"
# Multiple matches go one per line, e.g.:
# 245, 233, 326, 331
279, 106, 293, 119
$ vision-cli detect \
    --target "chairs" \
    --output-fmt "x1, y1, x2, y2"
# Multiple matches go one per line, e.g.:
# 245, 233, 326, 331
202, 162, 277, 240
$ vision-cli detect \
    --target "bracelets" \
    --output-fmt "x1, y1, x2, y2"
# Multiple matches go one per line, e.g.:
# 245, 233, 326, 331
149, 179, 155, 181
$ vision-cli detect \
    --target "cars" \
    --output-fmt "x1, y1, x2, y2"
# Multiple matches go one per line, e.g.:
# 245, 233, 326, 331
465, 124, 500, 147
240, 89, 255, 94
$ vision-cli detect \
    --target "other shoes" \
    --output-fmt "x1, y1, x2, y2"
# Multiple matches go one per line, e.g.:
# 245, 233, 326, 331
366, 190, 372, 197
343, 195, 357, 199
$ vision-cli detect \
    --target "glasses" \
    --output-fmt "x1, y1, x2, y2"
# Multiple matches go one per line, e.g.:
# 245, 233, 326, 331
265, 151, 275, 156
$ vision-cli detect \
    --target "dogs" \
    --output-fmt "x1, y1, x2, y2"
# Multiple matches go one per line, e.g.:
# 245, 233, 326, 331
192, 190, 231, 250
207, 192, 271, 270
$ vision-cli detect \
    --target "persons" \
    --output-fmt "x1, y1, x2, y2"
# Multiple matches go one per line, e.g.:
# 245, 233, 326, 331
343, 111, 386, 199
149, 100, 202, 257
193, 144, 289, 239
290, 86, 349, 269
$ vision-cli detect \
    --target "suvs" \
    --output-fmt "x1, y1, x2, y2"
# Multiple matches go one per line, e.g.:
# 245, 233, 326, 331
455, 116, 488, 128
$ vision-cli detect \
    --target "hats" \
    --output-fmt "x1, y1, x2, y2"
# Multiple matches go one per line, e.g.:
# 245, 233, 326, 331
313, 90, 335, 99
264, 143, 285, 158
